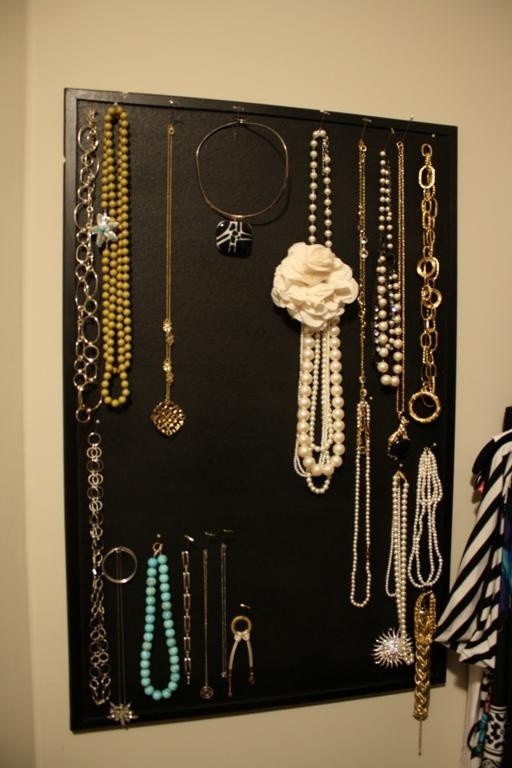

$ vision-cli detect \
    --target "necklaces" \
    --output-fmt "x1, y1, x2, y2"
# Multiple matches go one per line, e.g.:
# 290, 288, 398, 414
73, 103, 443, 757
